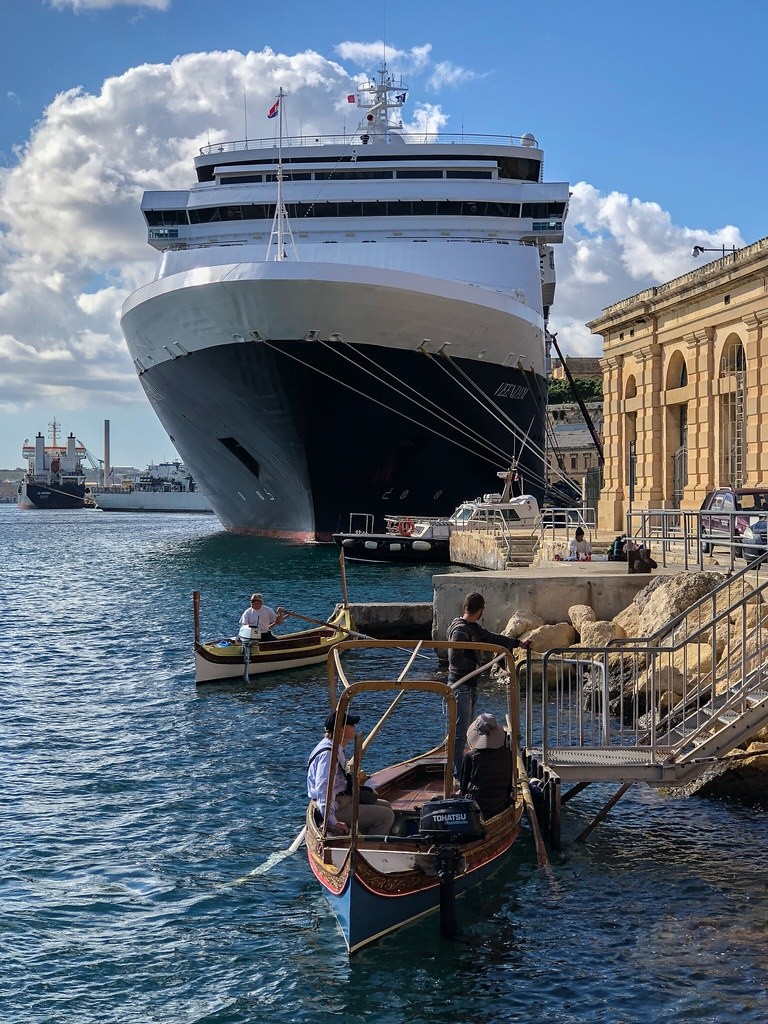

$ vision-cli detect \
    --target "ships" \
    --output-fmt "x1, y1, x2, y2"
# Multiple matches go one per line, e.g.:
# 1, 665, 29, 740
120, 27, 569, 544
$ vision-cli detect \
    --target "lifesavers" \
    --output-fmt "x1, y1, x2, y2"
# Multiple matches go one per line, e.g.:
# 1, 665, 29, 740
397, 519, 415, 535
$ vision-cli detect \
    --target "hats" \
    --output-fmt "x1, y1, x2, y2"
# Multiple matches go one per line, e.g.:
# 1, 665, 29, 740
463, 592, 484, 610
466, 712, 505, 750
576, 526, 584, 536
249, 593, 263, 600
323, 710, 360, 733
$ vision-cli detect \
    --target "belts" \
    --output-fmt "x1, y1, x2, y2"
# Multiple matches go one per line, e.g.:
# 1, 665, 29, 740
336, 790, 350, 797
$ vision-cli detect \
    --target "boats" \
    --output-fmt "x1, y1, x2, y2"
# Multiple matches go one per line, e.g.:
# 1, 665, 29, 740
193, 547, 357, 684
288, 640, 550, 956
90, 459, 213, 511
18, 416, 90, 510
331, 417, 573, 565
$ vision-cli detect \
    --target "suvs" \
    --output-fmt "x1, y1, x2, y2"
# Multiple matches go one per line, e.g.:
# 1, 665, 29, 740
698, 486, 768, 552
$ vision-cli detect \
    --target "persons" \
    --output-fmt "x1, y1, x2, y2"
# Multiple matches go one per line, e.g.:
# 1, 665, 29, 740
460, 712, 515, 821
307, 711, 395, 841
239, 594, 283, 642
568, 527, 592, 557
442, 592, 535, 795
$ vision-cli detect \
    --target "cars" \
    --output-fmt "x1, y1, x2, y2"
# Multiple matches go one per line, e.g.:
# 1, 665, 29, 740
742, 514, 768, 570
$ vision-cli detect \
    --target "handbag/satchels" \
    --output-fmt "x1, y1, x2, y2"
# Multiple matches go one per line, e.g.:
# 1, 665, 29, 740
348, 786, 378, 804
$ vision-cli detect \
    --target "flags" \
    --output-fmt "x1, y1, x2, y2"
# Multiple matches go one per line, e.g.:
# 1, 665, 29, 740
267, 98, 279, 118
347, 94, 355, 103
395, 93, 406, 103
